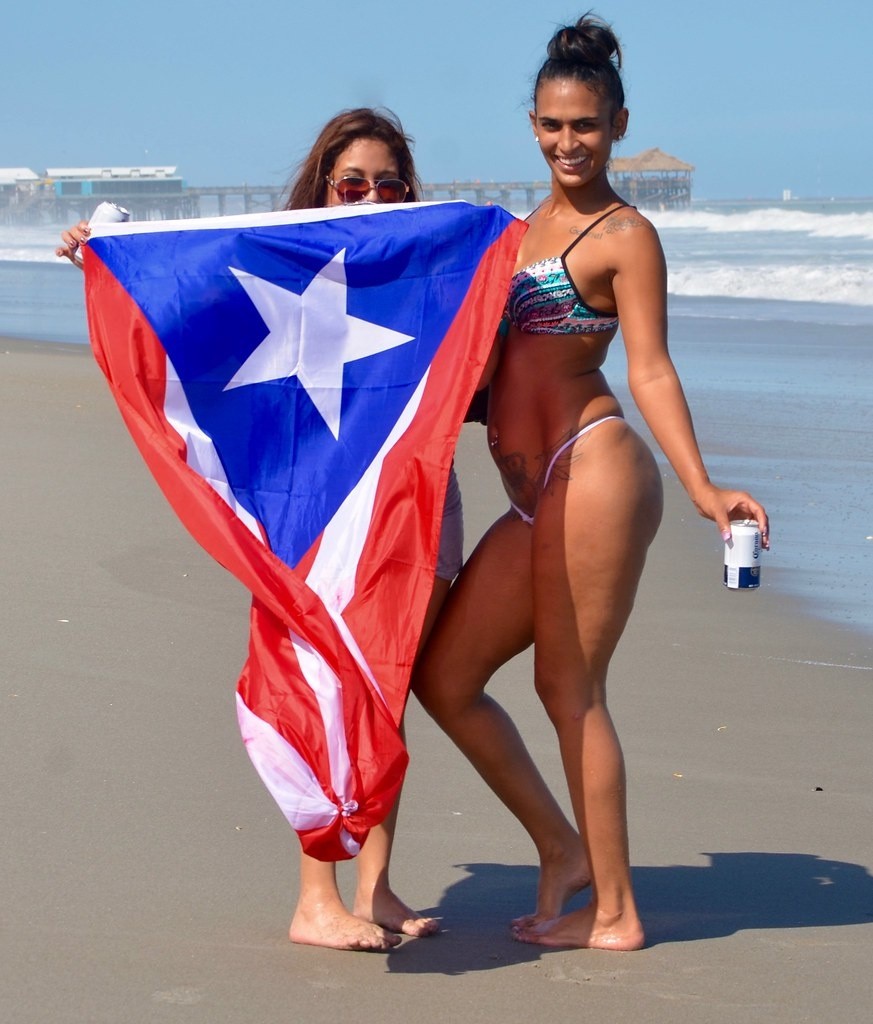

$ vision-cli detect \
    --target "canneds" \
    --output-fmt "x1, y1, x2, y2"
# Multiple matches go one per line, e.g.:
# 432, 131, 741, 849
73, 201, 130, 265
722, 519, 763, 591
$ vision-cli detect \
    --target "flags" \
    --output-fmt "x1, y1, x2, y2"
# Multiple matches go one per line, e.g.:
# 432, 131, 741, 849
80, 200, 530, 861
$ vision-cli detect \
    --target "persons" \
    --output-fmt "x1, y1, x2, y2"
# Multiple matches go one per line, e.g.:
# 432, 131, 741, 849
56, 108, 491, 950
412, 15, 770, 952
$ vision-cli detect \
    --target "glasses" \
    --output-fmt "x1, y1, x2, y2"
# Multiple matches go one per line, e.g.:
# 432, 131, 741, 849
326, 175, 410, 203
